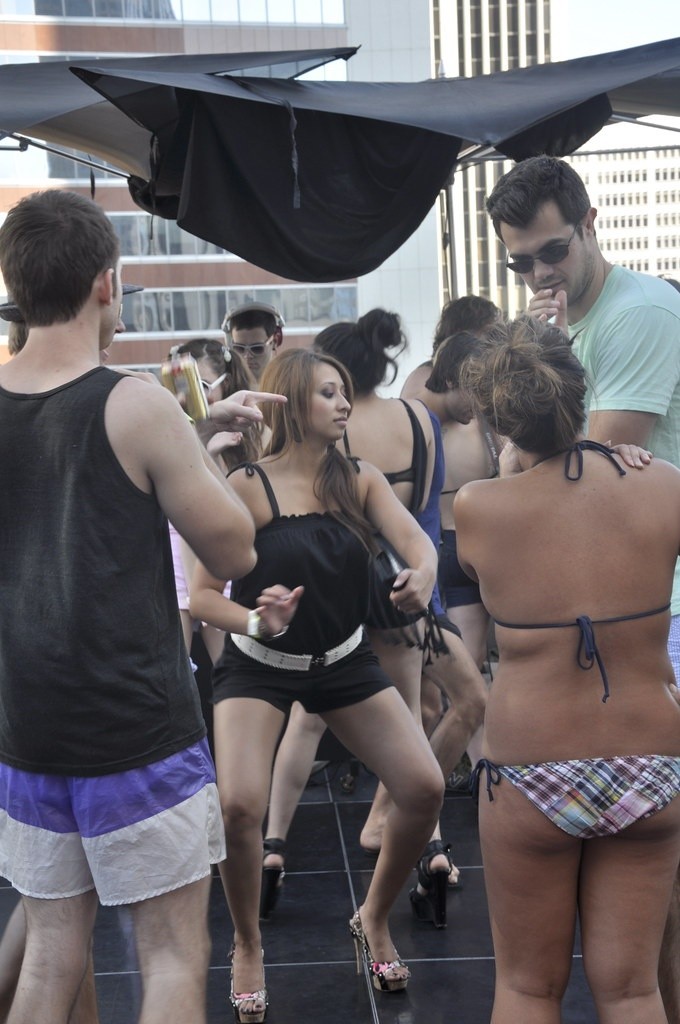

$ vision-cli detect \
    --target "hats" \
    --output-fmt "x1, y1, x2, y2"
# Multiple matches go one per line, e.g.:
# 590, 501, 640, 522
122, 284, 143, 295
0, 303, 25, 322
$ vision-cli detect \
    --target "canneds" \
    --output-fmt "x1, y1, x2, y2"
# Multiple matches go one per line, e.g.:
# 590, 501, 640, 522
159, 352, 210, 422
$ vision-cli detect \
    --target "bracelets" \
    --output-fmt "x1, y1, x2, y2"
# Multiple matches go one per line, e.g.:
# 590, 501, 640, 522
248, 607, 289, 639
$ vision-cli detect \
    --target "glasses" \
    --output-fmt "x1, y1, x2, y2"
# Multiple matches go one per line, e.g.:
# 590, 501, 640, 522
505, 220, 577, 273
233, 335, 274, 356
118, 304, 123, 318
200, 373, 227, 397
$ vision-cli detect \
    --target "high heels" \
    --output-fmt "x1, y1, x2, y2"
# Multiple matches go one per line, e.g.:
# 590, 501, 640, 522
227, 942, 269, 1024
409, 839, 452, 928
260, 837, 286, 920
349, 906, 411, 991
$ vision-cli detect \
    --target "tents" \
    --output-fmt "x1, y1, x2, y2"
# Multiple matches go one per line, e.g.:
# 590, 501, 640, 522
1, 35, 679, 284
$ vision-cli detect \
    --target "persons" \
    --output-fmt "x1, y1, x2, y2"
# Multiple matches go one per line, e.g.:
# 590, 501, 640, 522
453, 317, 680, 1024
0, 188, 257, 1023
2, 282, 510, 1023
486, 156, 680, 1023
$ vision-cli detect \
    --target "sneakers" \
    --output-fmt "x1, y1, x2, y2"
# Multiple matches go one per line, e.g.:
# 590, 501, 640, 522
445, 762, 474, 791
